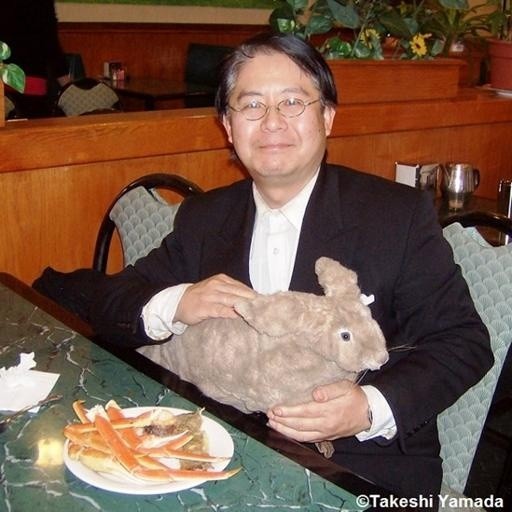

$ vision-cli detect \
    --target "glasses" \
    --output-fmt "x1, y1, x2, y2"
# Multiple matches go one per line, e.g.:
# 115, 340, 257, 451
223, 97, 324, 122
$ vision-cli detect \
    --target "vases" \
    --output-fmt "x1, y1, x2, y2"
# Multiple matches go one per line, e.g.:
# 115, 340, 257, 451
320, 57, 466, 103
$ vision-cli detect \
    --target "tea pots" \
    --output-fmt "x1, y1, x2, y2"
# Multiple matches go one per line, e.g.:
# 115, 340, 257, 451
439, 159, 481, 212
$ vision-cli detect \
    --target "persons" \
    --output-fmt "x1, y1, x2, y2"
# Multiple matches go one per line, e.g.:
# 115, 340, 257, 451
74, 33, 493, 511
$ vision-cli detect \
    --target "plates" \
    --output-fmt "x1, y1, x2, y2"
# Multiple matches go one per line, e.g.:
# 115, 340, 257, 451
60, 405, 236, 497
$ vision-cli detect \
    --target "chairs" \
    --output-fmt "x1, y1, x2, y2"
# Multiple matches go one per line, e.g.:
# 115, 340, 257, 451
90, 173, 207, 276
53, 78, 121, 114
434, 213, 511, 510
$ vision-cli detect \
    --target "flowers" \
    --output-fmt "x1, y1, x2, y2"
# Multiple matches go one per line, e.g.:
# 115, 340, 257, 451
266, 0, 510, 59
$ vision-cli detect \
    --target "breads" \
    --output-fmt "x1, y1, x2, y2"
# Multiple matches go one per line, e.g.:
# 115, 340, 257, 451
144, 413, 214, 471
